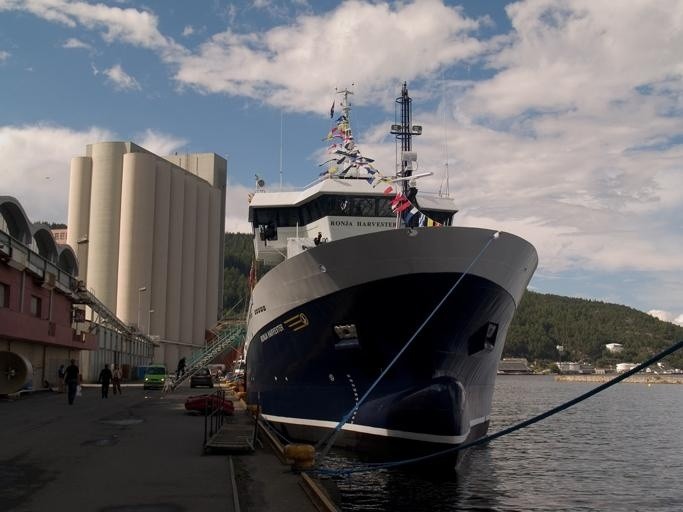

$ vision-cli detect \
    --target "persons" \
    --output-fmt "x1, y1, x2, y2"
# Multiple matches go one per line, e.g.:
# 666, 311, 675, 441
175, 357, 186, 379
98, 363, 111, 400
111, 365, 122, 395
62, 359, 79, 405
57, 364, 65, 393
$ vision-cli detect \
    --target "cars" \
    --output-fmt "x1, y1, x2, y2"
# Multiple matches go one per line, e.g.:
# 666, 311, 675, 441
189, 363, 225, 390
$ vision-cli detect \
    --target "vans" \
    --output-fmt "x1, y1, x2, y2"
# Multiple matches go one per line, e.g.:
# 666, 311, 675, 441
142, 363, 168, 390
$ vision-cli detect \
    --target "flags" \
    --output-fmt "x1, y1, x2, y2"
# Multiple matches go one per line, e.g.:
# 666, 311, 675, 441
318, 98, 443, 231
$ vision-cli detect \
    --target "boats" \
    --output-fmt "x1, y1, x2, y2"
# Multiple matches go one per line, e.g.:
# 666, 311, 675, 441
237, 80, 543, 479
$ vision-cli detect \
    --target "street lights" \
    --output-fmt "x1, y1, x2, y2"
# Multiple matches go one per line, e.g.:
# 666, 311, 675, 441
136, 286, 146, 331
146, 309, 155, 339
70, 235, 88, 281
